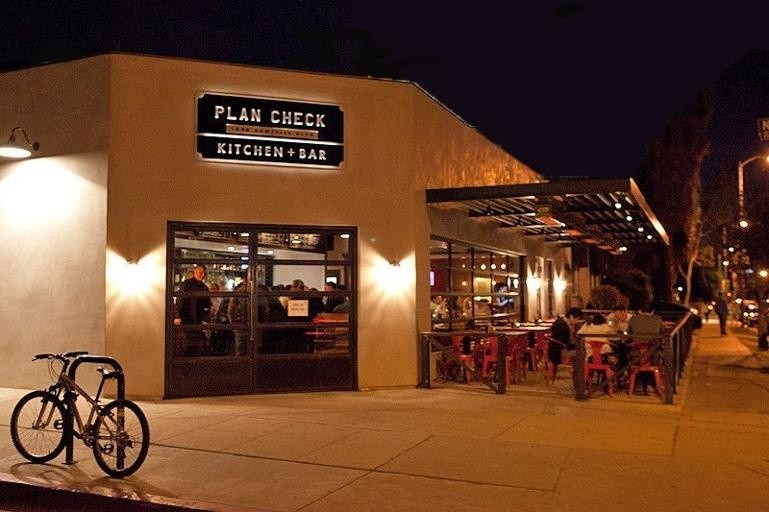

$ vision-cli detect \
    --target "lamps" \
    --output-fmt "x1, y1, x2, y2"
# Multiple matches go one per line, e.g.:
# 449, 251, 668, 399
0, 126, 41, 160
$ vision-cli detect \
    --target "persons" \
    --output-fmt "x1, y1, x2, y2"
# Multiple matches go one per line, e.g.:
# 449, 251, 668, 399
606, 304, 633, 375
582, 314, 621, 393
429, 281, 513, 326
549, 307, 588, 385
714, 292, 731, 334
172, 264, 348, 357
626, 304, 665, 392
579, 314, 595, 329
702, 301, 709, 322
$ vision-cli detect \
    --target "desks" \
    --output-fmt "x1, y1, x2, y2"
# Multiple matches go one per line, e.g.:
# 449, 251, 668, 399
471, 319, 555, 385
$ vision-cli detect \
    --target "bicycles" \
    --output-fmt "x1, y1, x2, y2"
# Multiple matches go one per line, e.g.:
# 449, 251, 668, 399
9, 348, 155, 480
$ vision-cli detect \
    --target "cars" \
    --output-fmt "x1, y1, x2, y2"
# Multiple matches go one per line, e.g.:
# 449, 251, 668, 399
733, 297, 768, 331
634, 300, 703, 332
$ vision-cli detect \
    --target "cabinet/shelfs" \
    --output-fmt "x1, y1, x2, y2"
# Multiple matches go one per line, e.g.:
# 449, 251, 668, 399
313, 313, 350, 353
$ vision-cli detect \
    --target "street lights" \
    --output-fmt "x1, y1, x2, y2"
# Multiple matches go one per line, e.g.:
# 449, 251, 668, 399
734, 154, 769, 220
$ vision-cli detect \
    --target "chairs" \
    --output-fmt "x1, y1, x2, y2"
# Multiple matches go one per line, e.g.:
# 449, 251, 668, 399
583, 340, 618, 398
444, 331, 549, 385
626, 341, 664, 399
